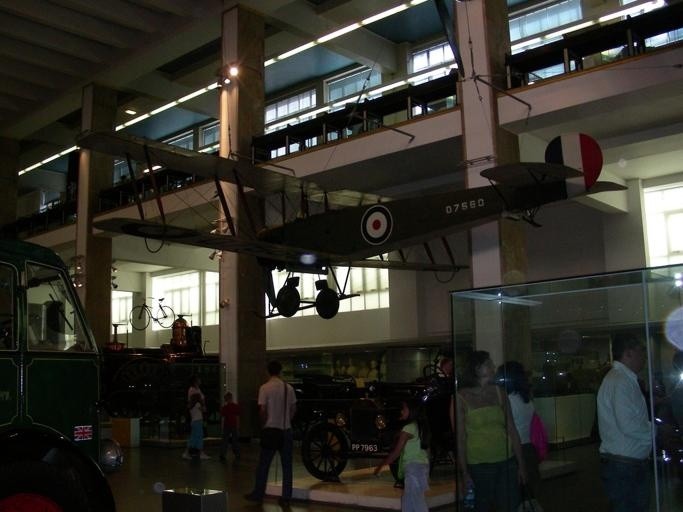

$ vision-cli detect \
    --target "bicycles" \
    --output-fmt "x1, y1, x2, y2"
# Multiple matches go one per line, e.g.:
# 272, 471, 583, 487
126, 295, 175, 333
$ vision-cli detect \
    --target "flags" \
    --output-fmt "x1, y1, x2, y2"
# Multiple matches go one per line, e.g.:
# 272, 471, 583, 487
73, 424, 92, 441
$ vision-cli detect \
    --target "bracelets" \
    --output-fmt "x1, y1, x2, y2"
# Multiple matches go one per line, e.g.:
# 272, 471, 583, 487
460, 472, 469, 475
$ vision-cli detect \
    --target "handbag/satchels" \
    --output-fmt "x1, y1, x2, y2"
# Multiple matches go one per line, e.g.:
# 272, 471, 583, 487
577, 441, 601, 480
529, 410, 548, 462
514, 476, 545, 512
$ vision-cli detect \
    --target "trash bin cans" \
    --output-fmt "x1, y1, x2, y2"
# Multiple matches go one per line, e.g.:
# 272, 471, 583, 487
162, 488, 227, 512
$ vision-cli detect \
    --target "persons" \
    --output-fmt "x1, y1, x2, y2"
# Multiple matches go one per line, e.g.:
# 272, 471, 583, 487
653, 376, 674, 432
218, 392, 242, 461
241, 360, 296, 509
594, 333, 673, 512
638, 375, 650, 421
451, 350, 528, 511
672, 350, 682, 479
180, 374, 210, 460
494, 360, 545, 511
439, 357, 454, 377
374, 398, 430, 511
187, 392, 204, 462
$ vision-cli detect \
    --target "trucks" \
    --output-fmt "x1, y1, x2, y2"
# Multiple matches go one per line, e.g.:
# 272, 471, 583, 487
0, 232, 118, 511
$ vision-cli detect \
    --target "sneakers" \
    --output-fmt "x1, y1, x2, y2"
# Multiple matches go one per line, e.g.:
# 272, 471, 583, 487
182, 451, 213, 460
242, 491, 290, 508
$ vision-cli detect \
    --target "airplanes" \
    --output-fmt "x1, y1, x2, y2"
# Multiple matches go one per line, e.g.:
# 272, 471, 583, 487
72, 125, 630, 321
